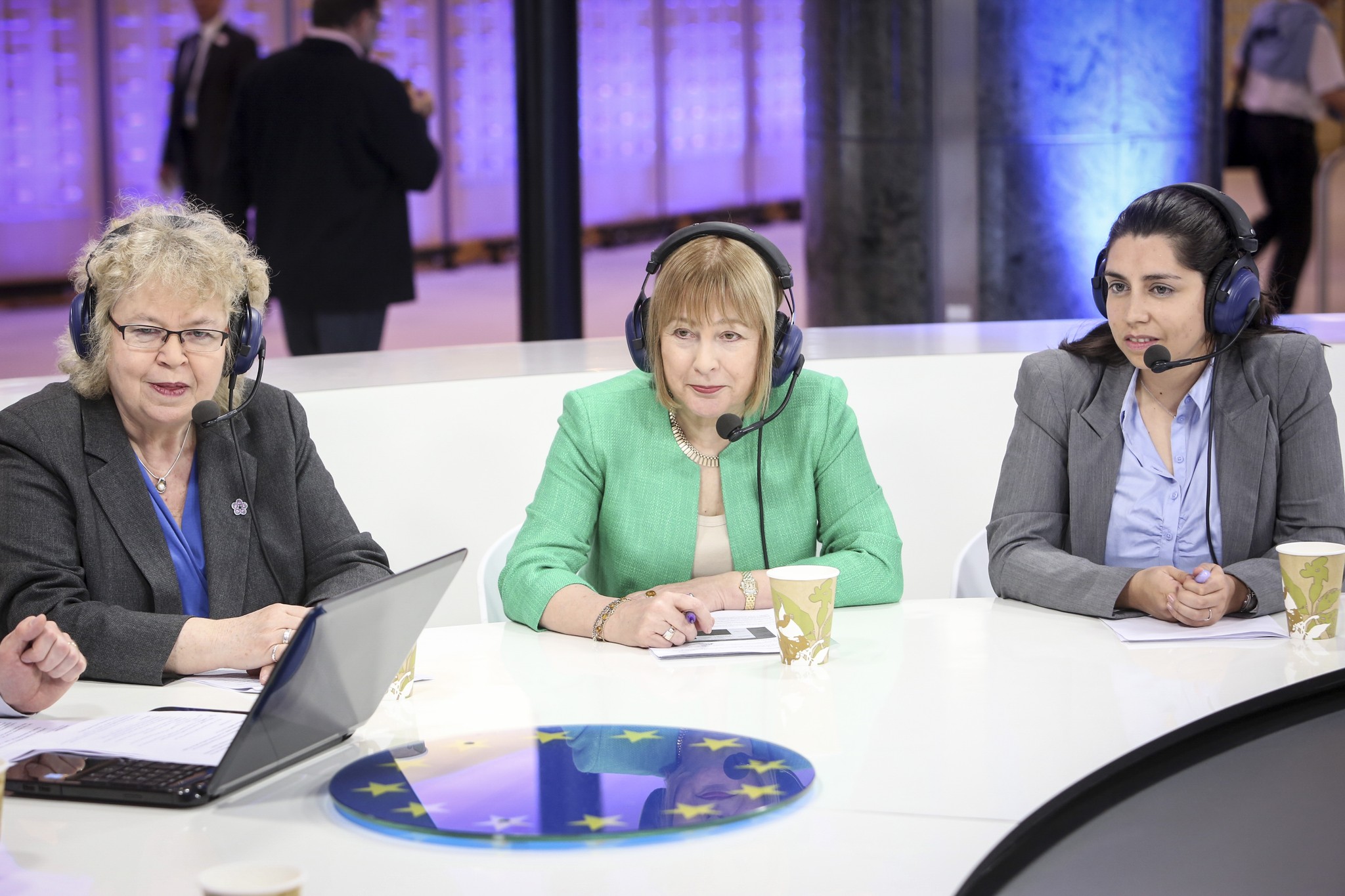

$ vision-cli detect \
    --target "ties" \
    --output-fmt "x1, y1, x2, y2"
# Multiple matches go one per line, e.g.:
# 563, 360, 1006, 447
178, 34, 200, 125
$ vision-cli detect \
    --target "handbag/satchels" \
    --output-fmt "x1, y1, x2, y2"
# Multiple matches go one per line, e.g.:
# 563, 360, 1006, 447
1221, 107, 1251, 165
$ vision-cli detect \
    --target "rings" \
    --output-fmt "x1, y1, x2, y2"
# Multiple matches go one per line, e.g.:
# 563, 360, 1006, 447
1203, 608, 1212, 621
644, 590, 656, 598
282, 628, 291, 644
272, 644, 279, 663
662, 626, 676, 641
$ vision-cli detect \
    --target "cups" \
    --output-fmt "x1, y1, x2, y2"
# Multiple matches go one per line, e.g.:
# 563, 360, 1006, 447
1276, 541, 1345, 640
382, 641, 417, 698
766, 565, 840, 667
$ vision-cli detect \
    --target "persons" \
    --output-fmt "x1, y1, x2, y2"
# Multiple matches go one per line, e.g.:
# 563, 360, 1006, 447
986, 186, 1345, 628
157, 0, 259, 243
0, 613, 88, 719
0, 188, 395, 689
1230, 0, 1345, 315
497, 234, 903, 649
244, 0, 440, 356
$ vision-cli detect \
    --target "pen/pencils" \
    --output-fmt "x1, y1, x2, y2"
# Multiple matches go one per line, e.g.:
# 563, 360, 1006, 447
1195, 570, 1209, 583
686, 593, 696, 623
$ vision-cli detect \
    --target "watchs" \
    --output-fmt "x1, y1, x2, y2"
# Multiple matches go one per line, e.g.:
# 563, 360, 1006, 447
737, 571, 759, 610
1237, 584, 1257, 613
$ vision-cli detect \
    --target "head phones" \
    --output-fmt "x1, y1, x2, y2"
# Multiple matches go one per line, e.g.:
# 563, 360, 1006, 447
639, 769, 805, 830
625, 221, 805, 387
69, 216, 266, 390
1091, 183, 1260, 350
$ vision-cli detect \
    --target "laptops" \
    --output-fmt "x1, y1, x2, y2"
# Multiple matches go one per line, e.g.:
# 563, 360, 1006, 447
3, 547, 468, 808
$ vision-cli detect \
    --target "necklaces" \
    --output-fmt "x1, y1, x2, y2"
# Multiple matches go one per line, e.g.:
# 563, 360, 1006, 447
667, 411, 721, 468
135, 421, 192, 496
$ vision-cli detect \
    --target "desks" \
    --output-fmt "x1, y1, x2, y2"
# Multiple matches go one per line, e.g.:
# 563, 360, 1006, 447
1, 602, 1345, 896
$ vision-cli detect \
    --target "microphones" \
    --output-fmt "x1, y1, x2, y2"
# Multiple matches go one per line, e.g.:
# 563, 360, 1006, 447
191, 358, 265, 428
723, 752, 805, 790
1143, 321, 1248, 373
716, 374, 797, 443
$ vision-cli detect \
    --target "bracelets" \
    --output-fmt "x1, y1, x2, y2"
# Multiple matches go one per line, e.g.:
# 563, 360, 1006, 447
592, 597, 633, 642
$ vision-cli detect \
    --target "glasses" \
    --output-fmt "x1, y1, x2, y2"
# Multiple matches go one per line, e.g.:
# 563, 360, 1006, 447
108, 311, 230, 352
